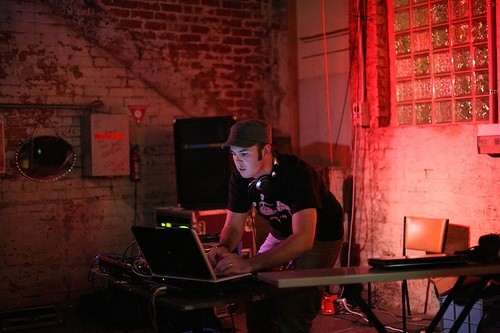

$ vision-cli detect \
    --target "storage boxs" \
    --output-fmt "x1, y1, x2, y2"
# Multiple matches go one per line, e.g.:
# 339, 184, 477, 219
155, 206, 258, 258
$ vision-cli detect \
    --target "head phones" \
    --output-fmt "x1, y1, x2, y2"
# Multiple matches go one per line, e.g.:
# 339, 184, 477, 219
247, 154, 280, 194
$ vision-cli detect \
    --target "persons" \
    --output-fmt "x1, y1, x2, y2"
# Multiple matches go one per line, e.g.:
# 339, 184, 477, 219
207, 119, 343, 333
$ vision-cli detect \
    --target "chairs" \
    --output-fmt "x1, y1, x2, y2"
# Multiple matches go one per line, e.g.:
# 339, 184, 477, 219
402, 216, 449, 333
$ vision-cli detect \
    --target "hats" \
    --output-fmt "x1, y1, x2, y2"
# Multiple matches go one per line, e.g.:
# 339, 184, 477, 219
225, 119, 272, 148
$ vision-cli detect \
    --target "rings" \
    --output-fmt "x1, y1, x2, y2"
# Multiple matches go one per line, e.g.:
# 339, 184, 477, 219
229, 263, 232, 267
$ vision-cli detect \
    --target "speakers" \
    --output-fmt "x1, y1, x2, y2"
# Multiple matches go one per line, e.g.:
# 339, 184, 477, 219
174, 115, 236, 211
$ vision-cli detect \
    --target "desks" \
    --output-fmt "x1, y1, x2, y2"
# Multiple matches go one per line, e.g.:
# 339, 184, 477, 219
89, 268, 500, 333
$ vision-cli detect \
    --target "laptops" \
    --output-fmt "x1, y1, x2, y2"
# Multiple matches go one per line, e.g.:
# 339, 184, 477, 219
130, 226, 252, 283
